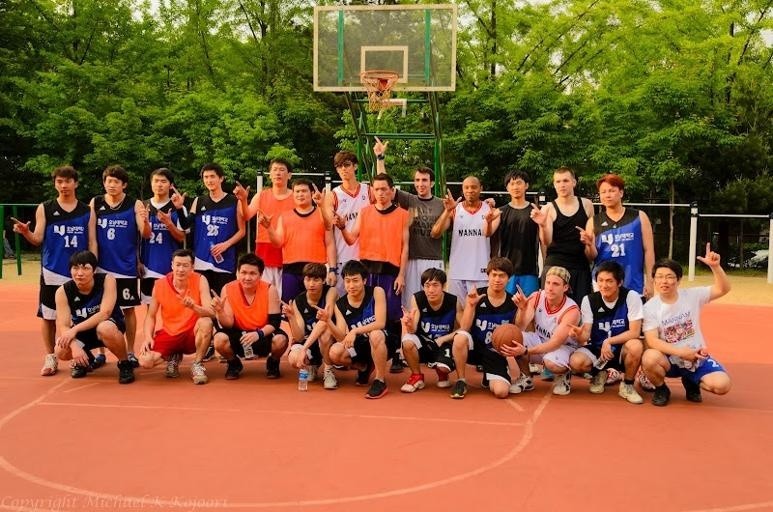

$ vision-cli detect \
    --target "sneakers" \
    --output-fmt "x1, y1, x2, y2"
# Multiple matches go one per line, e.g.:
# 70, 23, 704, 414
117, 361, 135, 383
365, 378, 389, 399
88, 354, 105, 372
482, 367, 490, 387
203, 345, 215, 362
324, 366, 339, 389
638, 371, 655, 390
355, 363, 375, 387
652, 384, 671, 405
128, 353, 139, 368
388, 351, 406, 373
590, 371, 608, 393
400, 374, 425, 393
606, 368, 621, 384
509, 375, 535, 393
225, 358, 244, 379
72, 352, 94, 378
552, 371, 571, 395
163, 352, 183, 377
541, 368, 553, 381
190, 359, 208, 384
41, 353, 57, 375
450, 378, 468, 399
307, 366, 320, 382
434, 364, 452, 388
681, 378, 703, 403
618, 381, 643, 404
266, 356, 280, 378
529, 363, 541, 376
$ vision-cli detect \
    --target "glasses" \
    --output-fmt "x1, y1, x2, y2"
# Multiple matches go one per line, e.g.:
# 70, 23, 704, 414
655, 273, 677, 281
336, 161, 355, 168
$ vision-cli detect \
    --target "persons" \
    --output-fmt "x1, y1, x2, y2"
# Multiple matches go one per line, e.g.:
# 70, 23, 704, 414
55, 249, 136, 384
331, 172, 412, 374
10, 165, 92, 377
310, 259, 396, 398
371, 135, 497, 368
528, 166, 595, 382
134, 166, 190, 351
575, 172, 658, 392
138, 248, 217, 384
256, 178, 338, 356
87, 165, 151, 366
484, 170, 542, 375
232, 156, 296, 356
311, 150, 373, 370
640, 242, 732, 406
430, 175, 493, 372
170, 162, 246, 363
278, 263, 341, 389
567, 260, 644, 403
449, 256, 519, 399
210, 252, 289, 381
400, 267, 463, 393
500, 265, 580, 396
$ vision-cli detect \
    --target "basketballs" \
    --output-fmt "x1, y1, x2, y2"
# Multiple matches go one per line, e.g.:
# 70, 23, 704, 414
491, 323, 524, 357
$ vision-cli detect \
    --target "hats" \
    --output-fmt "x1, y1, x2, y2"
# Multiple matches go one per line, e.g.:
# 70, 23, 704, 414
547, 266, 574, 295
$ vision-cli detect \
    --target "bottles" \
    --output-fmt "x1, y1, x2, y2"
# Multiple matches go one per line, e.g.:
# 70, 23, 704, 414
242, 330, 254, 359
347, 347, 357, 358
298, 364, 308, 391
594, 346, 617, 370
209, 241, 224, 264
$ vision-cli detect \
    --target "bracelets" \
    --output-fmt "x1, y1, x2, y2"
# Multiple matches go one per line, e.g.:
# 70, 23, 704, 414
339, 225, 346, 230
525, 345, 529, 356
257, 328, 264, 341
376, 152, 385, 161
